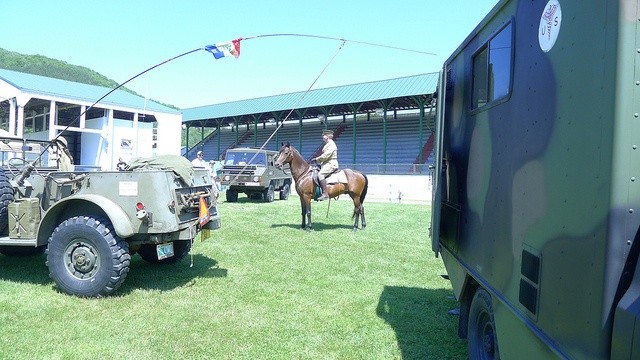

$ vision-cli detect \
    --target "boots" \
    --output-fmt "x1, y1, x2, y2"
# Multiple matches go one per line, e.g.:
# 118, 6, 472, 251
317, 179, 328, 200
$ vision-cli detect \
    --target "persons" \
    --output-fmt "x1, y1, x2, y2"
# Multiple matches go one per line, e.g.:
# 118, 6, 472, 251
311, 129, 340, 200
191, 151, 208, 168
208, 160, 217, 182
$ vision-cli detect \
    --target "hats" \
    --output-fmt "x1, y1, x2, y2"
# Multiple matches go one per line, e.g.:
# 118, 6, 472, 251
322, 130, 334, 134
197, 150, 204, 154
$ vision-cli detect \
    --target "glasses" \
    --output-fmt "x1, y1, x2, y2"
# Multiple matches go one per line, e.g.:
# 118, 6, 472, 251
199, 155, 204, 156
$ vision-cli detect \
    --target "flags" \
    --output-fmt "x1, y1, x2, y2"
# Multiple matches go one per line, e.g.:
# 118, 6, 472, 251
204, 38, 240, 60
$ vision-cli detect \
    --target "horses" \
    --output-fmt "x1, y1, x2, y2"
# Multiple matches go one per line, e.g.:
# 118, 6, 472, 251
275, 143, 368, 232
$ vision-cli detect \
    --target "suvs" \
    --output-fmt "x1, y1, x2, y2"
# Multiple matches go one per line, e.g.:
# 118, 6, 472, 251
429, 0, 639, 360
0, 136, 219, 296
220, 148, 293, 202
